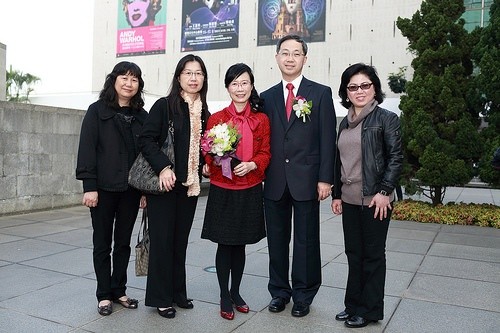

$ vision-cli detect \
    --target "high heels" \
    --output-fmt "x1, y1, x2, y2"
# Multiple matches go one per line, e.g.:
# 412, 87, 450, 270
230, 291, 250, 313
221, 307, 234, 320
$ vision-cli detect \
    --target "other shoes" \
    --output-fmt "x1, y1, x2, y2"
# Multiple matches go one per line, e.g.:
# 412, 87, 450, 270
176, 298, 193, 309
156, 306, 175, 318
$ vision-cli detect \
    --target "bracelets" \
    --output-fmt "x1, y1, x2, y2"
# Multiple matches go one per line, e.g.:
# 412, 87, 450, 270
379, 190, 389, 196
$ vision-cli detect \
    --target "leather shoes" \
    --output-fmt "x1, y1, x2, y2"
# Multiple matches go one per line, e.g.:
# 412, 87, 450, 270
291, 304, 309, 317
113, 296, 138, 309
98, 301, 112, 315
270, 296, 290, 312
345, 314, 378, 327
335, 308, 356, 320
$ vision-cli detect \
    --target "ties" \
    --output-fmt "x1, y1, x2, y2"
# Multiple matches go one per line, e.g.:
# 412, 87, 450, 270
286, 83, 295, 122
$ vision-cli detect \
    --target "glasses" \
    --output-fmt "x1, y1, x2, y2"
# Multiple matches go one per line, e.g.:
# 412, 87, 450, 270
347, 82, 373, 92
181, 71, 205, 77
278, 52, 304, 58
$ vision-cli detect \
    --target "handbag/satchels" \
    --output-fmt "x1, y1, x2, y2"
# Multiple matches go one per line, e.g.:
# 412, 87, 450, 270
127, 97, 175, 194
136, 207, 149, 276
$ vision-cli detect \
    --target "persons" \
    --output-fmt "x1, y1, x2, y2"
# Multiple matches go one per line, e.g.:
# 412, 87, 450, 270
203, 63, 271, 320
256, 34, 337, 317
75, 61, 149, 315
138, 54, 210, 319
330, 63, 404, 328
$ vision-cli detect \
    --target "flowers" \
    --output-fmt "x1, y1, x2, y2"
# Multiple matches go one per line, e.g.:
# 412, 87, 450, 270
198, 117, 241, 180
290, 95, 313, 122
